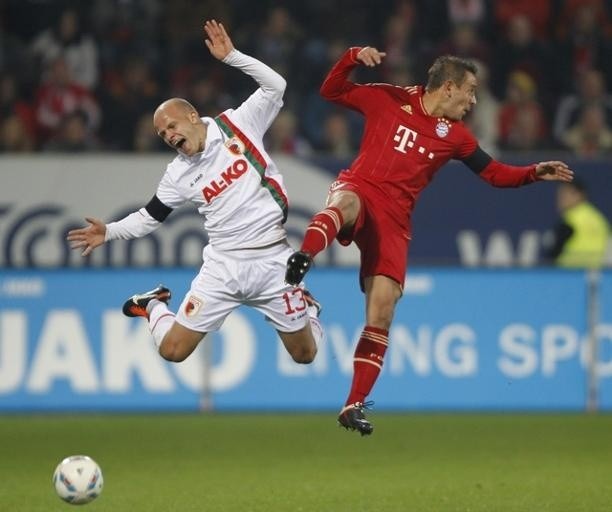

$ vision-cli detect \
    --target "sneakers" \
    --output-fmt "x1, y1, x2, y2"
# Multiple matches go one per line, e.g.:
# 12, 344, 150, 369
122, 284, 172, 319
283, 249, 311, 287
335, 402, 373, 436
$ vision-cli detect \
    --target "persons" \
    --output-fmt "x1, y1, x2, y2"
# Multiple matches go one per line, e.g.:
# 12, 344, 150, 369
0, 0, 612, 157
284, 45, 575, 437
537, 173, 611, 271
65, 17, 317, 364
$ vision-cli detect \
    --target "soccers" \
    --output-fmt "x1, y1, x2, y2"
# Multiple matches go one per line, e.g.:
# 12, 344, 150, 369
53, 455, 103, 504
